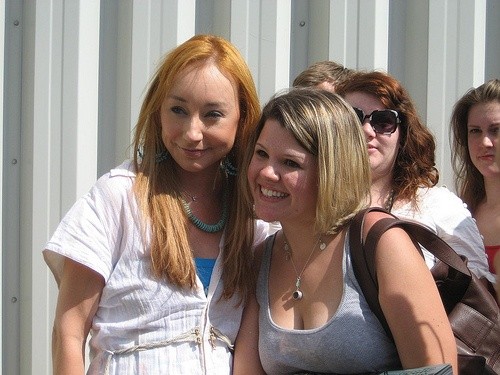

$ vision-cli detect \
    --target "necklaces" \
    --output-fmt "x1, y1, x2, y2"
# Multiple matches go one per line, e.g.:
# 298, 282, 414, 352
281, 229, 328, 299
169, 183, 228, 230
385, 188, 395, 213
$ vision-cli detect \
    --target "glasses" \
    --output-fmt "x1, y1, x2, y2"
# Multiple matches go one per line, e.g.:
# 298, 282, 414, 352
352, 105, 402, 135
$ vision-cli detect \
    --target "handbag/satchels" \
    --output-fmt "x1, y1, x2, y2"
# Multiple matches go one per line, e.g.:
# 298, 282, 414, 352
350, 207, 500, 375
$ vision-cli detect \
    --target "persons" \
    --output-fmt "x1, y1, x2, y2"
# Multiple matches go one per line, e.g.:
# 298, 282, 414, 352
231, 86, 459, 375
42, 35, 281, 375
292, 61, 500, 306
